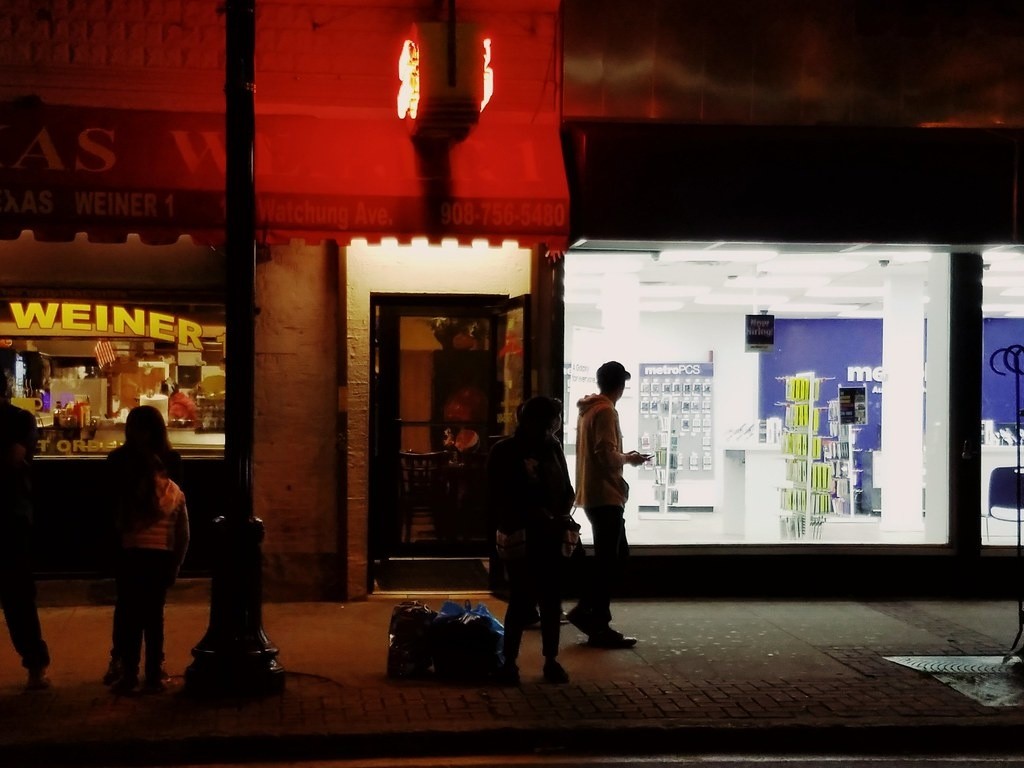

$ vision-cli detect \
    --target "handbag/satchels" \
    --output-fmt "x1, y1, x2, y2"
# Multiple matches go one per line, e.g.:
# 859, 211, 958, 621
387, 599, 505, 689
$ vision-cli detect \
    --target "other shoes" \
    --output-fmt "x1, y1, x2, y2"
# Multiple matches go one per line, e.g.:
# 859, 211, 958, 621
525, 615, 540, 626
103, 671, 116, 684
111, 679, 132, 696
501, 665, 520, 689
565, 607, 624, 645
542, 662, 569, 684
161, 671, 170, 680
25, 668, 50, 694
559, 611, 568, 621
139, 679, 167, 693
587, 636, 638, 649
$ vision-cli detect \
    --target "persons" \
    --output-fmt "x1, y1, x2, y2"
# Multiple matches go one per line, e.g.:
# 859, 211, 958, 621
482, 396, 576, 684
565, 361, 650, 648
88, 406, 191, 695
0, 370, 50, 691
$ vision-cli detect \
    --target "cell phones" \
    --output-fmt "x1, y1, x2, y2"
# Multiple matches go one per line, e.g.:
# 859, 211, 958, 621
646, 455, 656, 459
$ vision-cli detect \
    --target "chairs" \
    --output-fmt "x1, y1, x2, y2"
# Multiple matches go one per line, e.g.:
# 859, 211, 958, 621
400, 450, 449, 544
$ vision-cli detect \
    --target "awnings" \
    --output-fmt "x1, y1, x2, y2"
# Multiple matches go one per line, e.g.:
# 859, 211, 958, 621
0, 105, 571, 264
560, 118, 1024, 245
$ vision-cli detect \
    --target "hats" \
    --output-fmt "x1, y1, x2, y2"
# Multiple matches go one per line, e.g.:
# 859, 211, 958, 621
597, 362, 630, 381
